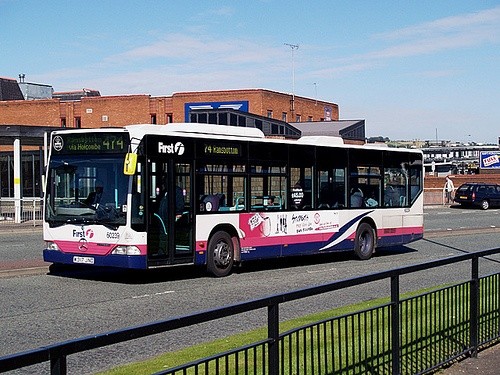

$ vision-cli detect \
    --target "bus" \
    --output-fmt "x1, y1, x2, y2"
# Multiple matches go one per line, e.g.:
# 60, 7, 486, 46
38, 120, 425, 278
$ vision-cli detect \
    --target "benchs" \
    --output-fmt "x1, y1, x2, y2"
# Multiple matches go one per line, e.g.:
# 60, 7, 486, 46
234, 195, 283, 210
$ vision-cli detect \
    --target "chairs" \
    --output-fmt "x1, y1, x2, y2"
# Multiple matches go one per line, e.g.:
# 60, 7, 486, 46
291, 185, 400, 209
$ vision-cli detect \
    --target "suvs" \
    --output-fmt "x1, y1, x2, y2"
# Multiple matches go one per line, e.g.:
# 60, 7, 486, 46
453, 182, 500, 210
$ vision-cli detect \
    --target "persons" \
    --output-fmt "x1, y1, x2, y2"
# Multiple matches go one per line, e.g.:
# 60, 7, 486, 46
157, 175, 185, 253
84, 179, 110, 206
443, 176, 455, 204
